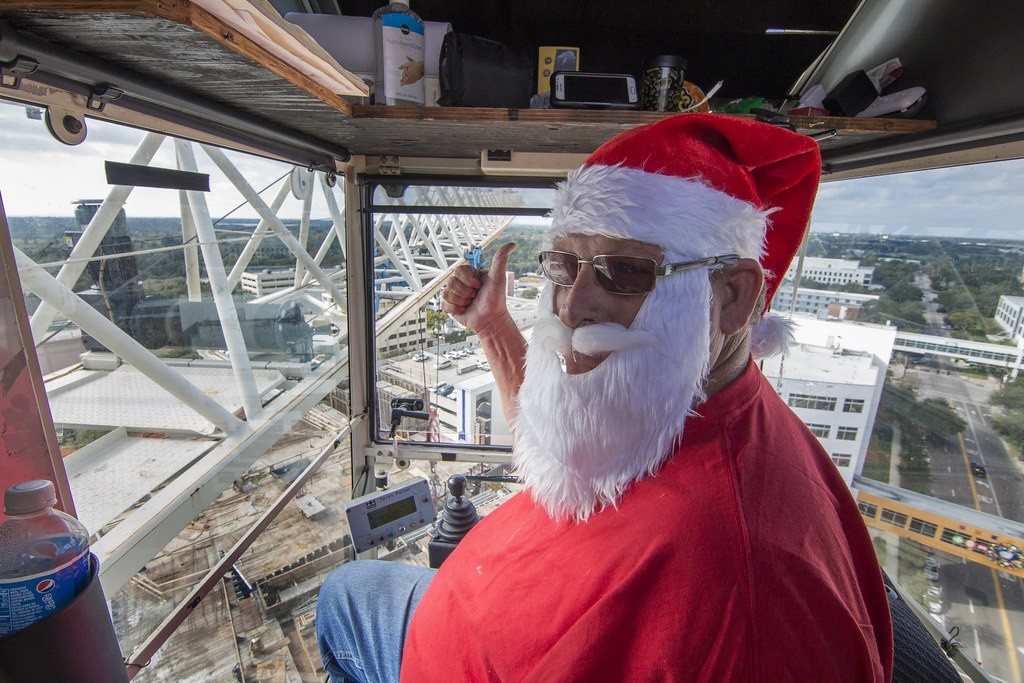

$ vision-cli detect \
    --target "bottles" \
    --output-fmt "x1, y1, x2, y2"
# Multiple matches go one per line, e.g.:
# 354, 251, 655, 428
372, 0, 425, 107
0, 479, 91, 635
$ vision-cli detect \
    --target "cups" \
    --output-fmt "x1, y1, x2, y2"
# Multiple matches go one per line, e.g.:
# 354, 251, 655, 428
641, 54, 688, 113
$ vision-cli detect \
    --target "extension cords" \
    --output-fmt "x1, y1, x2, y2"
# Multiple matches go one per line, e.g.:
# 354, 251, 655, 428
859, 86, 929, 118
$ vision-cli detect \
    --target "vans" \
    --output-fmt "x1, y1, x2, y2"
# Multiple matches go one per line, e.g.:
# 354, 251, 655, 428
434, 359, 452, 370
428, 381, 458, 400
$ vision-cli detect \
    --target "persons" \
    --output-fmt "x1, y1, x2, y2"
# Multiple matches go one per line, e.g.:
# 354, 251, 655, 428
315, 114, 893, 683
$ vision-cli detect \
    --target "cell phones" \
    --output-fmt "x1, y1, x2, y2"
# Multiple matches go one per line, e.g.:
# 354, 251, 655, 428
549, 71, 641, 109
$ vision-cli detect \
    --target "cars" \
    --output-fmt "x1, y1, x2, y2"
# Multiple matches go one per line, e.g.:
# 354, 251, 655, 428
922, 556, 939, 581
970, 461, 986, 478
912, 357, 926, 364
923, 586, 943, 614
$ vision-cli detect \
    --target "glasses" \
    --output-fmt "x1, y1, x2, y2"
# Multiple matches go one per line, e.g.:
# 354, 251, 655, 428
539, 250, 741, 295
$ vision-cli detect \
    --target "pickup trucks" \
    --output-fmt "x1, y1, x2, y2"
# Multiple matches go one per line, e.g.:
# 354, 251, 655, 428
477, 360, 490, 371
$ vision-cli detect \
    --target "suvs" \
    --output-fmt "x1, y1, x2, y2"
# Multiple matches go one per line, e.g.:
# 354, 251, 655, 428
413, 354, 429, 362
442, 347, 474, 360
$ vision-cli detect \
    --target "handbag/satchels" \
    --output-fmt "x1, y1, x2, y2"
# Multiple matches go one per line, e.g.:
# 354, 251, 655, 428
438, 32, 533, 108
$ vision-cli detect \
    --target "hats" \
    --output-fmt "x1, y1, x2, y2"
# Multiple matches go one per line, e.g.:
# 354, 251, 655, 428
549, 113, 820, 359
